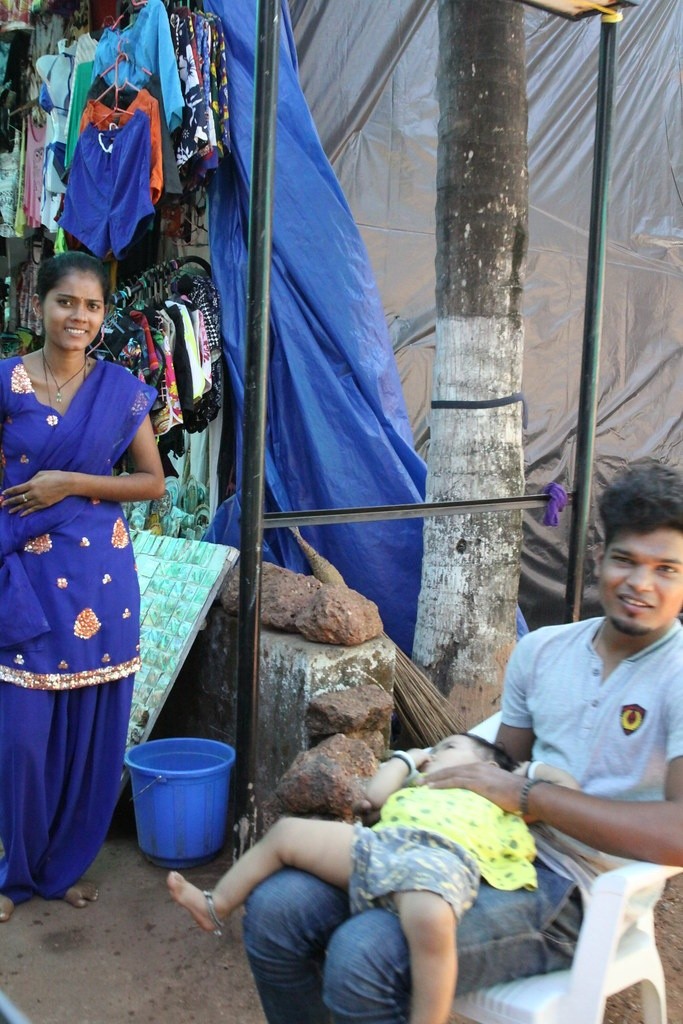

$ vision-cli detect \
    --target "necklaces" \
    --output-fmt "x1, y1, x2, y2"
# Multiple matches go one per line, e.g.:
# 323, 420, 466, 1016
43, 357, 55, 432
41, 346, 88, 402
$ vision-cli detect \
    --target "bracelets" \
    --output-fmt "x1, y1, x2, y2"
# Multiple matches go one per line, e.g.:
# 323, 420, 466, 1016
389, 750, 419, 785
519, 779, 552, 813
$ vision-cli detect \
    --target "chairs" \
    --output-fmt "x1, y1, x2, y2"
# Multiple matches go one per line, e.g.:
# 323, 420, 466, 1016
447, 712, 683, 1024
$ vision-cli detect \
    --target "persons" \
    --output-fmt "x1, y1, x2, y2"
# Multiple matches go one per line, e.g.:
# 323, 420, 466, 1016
239, 462, 681, 1024
1, 252, 166, 922
165, 727, 582, 1022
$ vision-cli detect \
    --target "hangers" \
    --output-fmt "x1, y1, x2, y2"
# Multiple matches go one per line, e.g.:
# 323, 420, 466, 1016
91, 258, 187, 357
93, 0, 200, 128
6, 113, 24, 142
30, 107, 47, 118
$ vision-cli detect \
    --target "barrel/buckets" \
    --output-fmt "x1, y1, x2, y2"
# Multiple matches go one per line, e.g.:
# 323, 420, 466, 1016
123, 737, 236, 869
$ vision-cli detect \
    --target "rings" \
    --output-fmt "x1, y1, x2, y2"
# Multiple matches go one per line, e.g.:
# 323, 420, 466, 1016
23, 495, 27, 502
